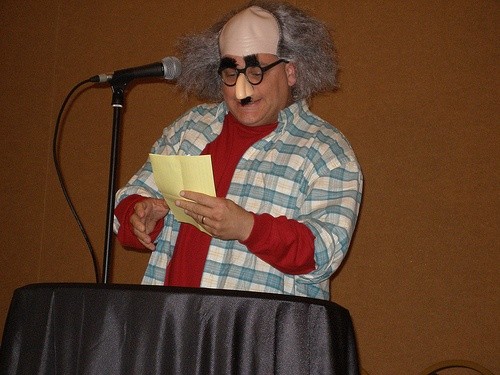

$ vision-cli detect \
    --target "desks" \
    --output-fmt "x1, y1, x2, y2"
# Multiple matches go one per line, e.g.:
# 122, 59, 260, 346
0, 283, 360, 375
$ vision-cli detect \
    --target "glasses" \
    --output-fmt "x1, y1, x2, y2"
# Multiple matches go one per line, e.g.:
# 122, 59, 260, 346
218, 58, 290, 88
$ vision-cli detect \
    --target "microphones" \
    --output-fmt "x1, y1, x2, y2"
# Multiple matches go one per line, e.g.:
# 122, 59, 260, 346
89, 56, 183, 83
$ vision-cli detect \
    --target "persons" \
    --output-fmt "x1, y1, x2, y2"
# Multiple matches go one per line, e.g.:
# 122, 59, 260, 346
111, 2, 364, 302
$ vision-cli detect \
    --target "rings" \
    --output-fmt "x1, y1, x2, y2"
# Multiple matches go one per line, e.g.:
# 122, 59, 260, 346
201, 217, 205, 224
